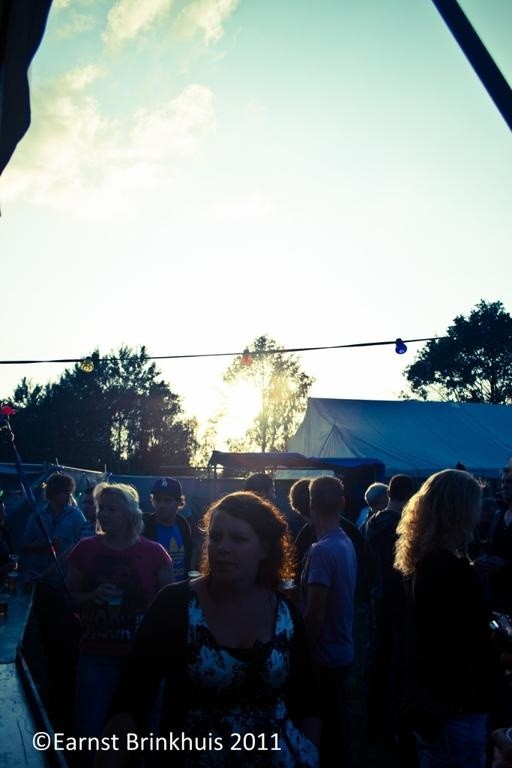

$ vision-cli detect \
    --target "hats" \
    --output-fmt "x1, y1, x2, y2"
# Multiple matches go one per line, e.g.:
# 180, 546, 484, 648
150, 477, 182, 498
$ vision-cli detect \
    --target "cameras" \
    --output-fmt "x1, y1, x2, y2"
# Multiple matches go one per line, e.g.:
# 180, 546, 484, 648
490, 614, 512, 636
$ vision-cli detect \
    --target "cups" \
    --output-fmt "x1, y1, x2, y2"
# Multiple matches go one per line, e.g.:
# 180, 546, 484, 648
0, 594, 9, 617
4, 576, 17, 593
23, 576, 32, 595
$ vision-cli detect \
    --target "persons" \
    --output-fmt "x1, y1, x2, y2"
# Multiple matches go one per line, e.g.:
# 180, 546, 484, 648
19, 461, 512, 767
0, 418, 15, 462
